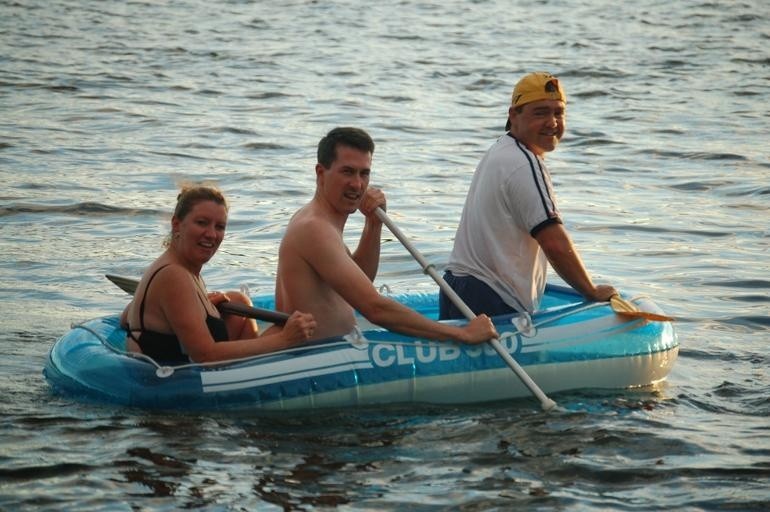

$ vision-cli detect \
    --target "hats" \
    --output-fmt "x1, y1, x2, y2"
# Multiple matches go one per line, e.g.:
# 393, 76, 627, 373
505, 73, 569, 131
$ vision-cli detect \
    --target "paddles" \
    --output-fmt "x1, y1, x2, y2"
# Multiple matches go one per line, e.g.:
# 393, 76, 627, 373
105, 274, 288, 329
545, 283, 673, 322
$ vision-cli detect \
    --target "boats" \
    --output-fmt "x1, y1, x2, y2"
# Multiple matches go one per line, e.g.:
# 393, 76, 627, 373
41, 269, 685, 416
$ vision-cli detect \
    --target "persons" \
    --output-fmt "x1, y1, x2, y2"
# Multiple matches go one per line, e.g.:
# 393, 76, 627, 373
119, 185, 317, 366
437, 72, 619, 319
275, 126, 498, 345
